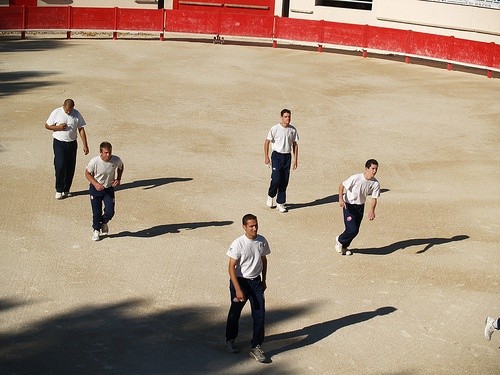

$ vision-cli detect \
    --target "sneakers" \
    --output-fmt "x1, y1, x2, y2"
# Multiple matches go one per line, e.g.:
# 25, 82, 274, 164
483, 316, 497, 341
248, 344, 266, 363
276, 203, 287, 212
267, 196, 273, 208
101, 223, 108, 235
55, 190, 72, 199
335, 235, 351, 255
224, 339, 239, 353
92, 229, 100, 241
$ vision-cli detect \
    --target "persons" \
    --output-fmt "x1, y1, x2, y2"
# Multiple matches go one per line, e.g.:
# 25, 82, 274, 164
85, 141, 124, 241
45, 99, 89, 200
225, 214, 271, 363
335, 158, 381, 256
483, 316, 500, 342
263, 108, 300, 213
